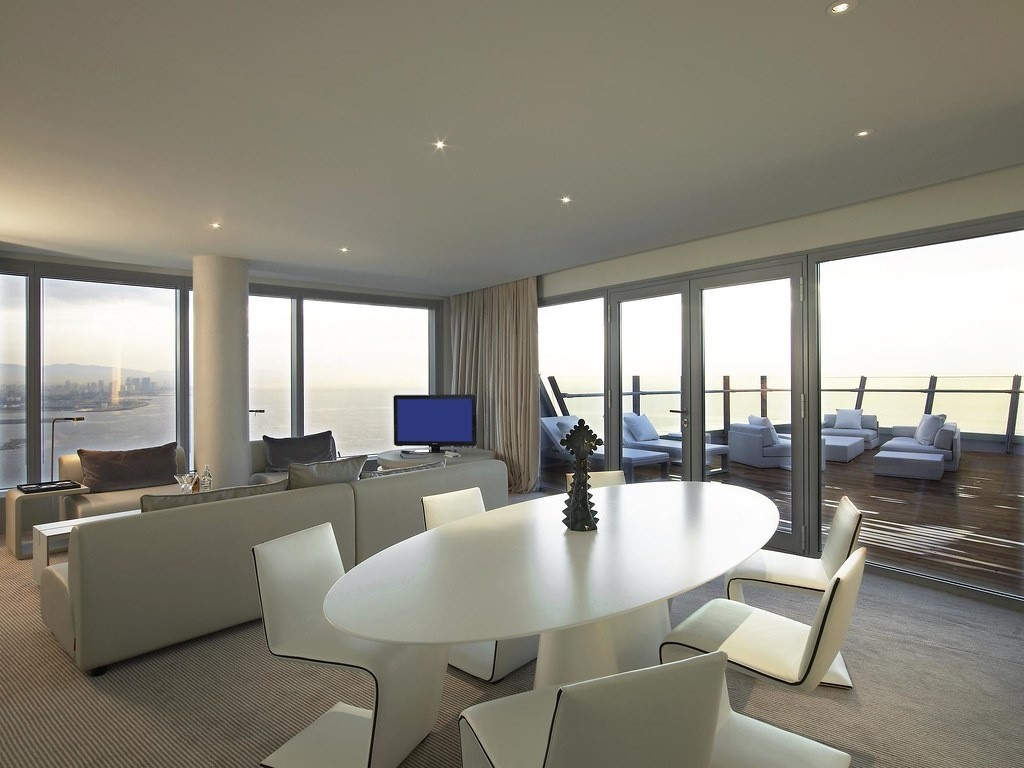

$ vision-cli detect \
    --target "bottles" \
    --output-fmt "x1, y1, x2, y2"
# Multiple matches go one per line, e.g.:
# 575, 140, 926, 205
201, 464, 212, 491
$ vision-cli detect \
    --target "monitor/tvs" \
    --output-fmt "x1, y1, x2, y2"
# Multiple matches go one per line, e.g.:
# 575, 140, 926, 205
392, 393, 477, 452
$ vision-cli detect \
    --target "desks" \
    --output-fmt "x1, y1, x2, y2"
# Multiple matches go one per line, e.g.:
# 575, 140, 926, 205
379, 448, 497, 470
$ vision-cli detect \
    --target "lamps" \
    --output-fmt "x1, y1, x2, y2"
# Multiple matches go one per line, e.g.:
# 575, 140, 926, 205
248, 409, 265, 413
49, 417, 85, 485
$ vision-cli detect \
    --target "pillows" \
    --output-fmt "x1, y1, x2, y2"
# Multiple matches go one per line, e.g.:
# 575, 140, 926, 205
263, 432, 340, 471
286, 454, 369, 492
556, 421, 578, 435
76, 441, 179, 492
835, 408, 864, 431
749, 416, 780, 446
914, 414, 947, 446
624, 414, 661, 441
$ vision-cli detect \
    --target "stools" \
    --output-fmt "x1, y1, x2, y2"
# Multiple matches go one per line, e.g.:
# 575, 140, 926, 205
822, 435, 864, 462
874, 451, 943, 479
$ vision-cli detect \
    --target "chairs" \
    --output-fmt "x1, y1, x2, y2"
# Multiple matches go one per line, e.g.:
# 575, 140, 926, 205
460, 651, 728, 767
540, 417, 670, 488
251, 521, 457, 768
420, 487, 539, 684
658, 546, 867, 768
603, 411, 729, 478
724, 494, 863, 691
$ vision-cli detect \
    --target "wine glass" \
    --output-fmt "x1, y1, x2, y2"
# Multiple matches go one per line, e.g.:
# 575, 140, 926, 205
174, 474, 186, 494
184, 473, 198, 493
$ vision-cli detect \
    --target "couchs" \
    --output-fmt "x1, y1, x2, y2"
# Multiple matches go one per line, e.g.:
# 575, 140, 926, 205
821, 414, 880, 451
248, 438, 339, 484
882, 426, 961, 474
41, 460, 511, 677
58, 446, 192, 520
729, 423, 791, 471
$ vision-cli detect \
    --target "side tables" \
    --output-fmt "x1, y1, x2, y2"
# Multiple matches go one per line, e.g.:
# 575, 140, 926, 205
31, 510, 143, 587
4, 480, 89, 560
